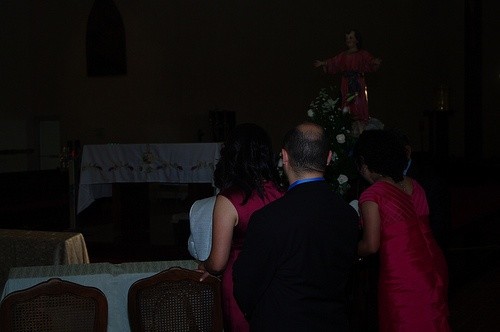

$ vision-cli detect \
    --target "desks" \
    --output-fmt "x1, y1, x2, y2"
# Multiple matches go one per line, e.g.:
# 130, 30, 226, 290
2, 260, 199, 332
67, 142, 223, 233
0, 229, 90, 266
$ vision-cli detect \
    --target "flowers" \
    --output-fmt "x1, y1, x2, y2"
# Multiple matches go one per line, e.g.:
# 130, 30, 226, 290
276, 92, 354, 203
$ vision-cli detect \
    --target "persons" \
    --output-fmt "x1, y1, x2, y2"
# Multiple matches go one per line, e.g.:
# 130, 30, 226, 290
198, 121, 288, 332
358, 129, 452, 332
234, 120, 363, 332
315, 28, 383, 122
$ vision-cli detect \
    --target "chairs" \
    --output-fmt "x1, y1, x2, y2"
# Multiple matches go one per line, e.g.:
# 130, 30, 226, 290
1, 279, 108, 332
128, 267, 223, 332
